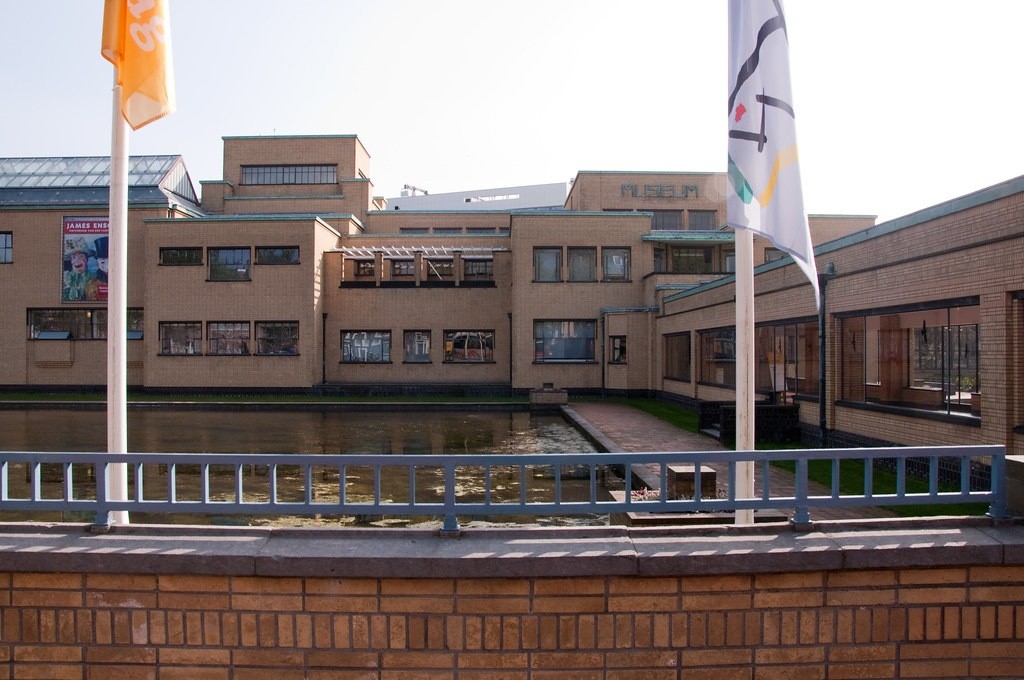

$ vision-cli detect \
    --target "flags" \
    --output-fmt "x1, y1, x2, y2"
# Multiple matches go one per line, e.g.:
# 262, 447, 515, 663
722, 0, 825, 316
101, 0, 174, 127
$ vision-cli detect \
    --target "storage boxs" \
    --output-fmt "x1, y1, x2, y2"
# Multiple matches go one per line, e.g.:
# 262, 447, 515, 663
665, 464, 716, 503
